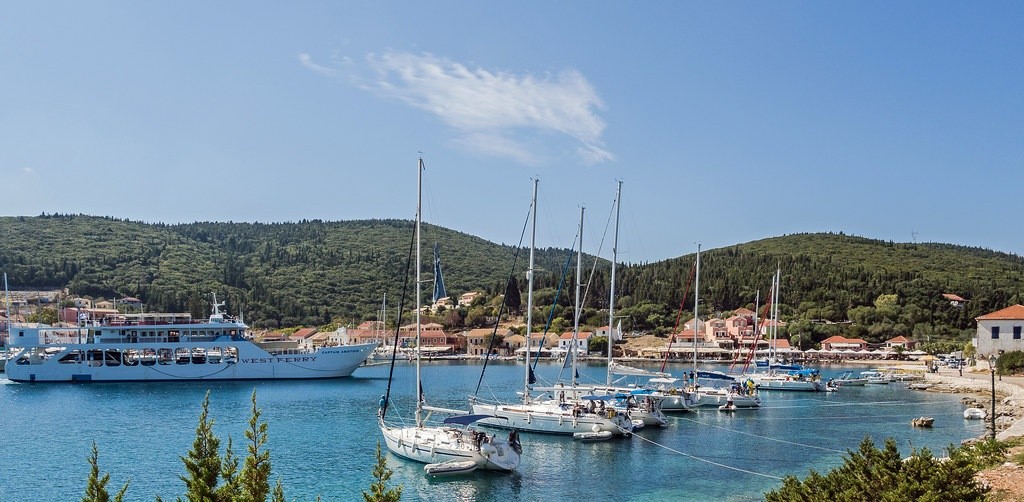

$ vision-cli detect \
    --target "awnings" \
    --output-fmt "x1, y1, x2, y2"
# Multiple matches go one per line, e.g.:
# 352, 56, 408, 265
641, 346, 929, 352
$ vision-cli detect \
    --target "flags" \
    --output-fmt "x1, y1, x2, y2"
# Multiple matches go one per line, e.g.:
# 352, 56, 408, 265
432, 242, 447, 305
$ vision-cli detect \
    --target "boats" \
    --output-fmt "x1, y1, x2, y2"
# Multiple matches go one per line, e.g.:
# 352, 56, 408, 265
378, 156, 523, 472
2, 272, 384, 382
963, 407, 987, 421
469, 175, 934, 439
423, 460, 479, 476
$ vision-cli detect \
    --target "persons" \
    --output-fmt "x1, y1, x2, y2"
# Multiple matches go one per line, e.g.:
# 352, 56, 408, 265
797, 370, 835, 390
96, 316, 112, 326
572, 369, 754, 420
376, 394, 389, 419
657, 353, 912, 362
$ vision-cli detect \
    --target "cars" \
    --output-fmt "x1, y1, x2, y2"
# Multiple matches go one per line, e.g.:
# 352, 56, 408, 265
934, 351, 967, 374
